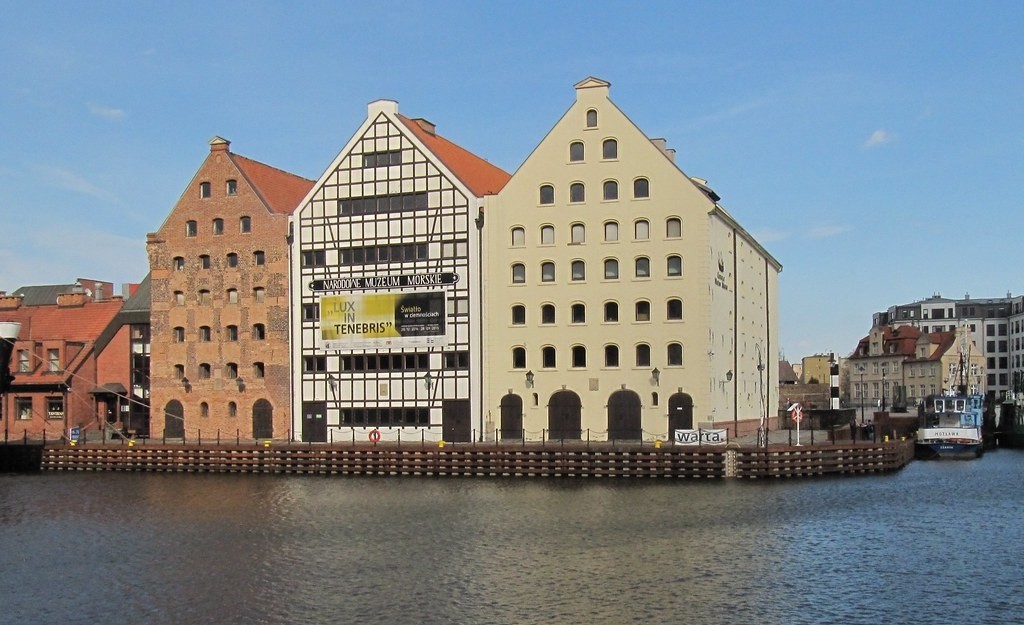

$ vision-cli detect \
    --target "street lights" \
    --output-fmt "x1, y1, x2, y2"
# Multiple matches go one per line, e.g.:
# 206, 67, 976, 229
881, 368, 886, 412
858, 366, 865, 423
828, 357, 836, 442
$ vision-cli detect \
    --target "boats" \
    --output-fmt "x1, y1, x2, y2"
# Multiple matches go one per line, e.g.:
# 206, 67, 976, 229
917, 319, 984, 458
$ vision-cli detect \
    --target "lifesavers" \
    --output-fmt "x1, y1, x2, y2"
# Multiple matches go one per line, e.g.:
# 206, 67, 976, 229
791, 409, 803, 423
369, 430, 381, 443
